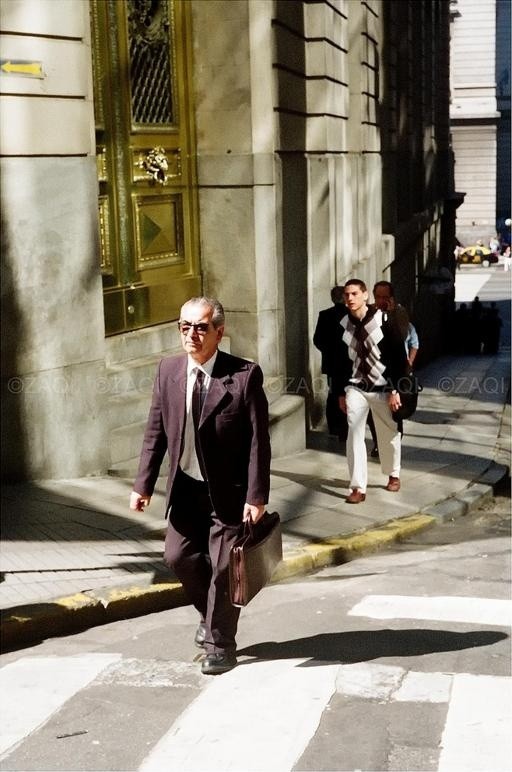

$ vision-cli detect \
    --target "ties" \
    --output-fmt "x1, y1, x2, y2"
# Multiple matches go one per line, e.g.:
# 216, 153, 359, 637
192, 369, 207, 482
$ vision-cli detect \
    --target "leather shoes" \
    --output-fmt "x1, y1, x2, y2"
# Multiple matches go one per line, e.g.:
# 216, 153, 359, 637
194, 622, 207, 648
201, 652, 238, 675
371, 447, 379, 457
345, 488, 366, 504
386, 475, 401, 492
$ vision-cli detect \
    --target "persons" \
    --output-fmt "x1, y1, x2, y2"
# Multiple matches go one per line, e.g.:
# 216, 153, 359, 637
311, 285, 354, 439
475, 232, 510, 257
338, 278, 402, 504
370, 280, 418, 459
127, 295, 274, 675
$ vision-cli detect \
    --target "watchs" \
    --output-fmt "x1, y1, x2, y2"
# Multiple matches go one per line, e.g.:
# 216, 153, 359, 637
388, 390, 398, 395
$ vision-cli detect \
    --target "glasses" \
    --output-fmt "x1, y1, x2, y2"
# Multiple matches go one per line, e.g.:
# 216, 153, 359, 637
178, 322, 209, 336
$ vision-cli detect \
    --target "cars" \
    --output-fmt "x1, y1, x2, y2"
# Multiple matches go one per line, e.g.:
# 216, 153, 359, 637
459, 247, 498, 267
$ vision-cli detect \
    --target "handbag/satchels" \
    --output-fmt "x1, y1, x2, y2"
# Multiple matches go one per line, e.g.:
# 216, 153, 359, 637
228, 509, 284, 608
391, 371, 419, 423
